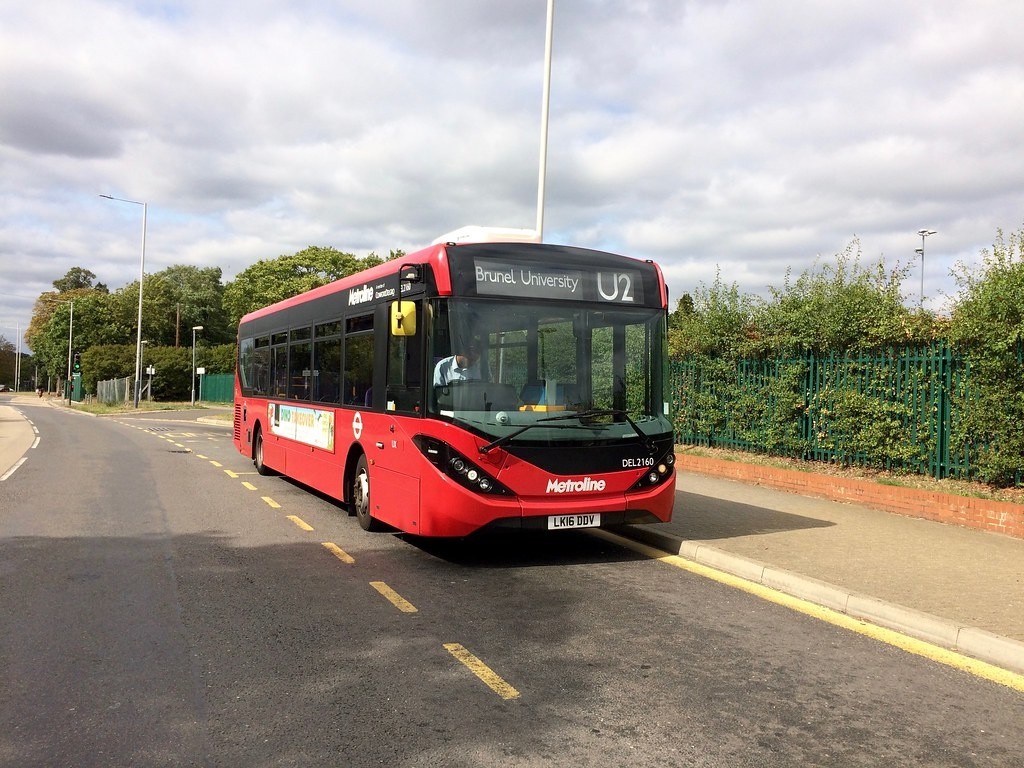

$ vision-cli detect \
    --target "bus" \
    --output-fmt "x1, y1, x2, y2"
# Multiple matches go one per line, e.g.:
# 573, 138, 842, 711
233, 226, 678, 540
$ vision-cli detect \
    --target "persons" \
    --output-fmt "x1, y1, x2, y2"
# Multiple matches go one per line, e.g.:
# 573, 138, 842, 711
433, 337, 483, 384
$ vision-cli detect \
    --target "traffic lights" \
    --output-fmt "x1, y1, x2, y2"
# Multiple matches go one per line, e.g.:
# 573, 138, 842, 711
74, 353, 80, 371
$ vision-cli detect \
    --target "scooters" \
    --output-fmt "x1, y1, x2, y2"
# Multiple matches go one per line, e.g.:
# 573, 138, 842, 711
38, 389, 43, 397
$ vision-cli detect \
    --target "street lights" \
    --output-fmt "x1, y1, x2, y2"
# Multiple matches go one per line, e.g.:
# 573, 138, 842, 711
38, 299, 73, 380
97, 193, 148, 409
191, 325, 203, 406
139, 340, 148, 401
5, 326, 21, 392
915, 228, 936, 308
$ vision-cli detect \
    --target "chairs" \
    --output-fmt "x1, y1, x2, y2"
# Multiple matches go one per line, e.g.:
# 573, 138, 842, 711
246, 353, 419, 413
